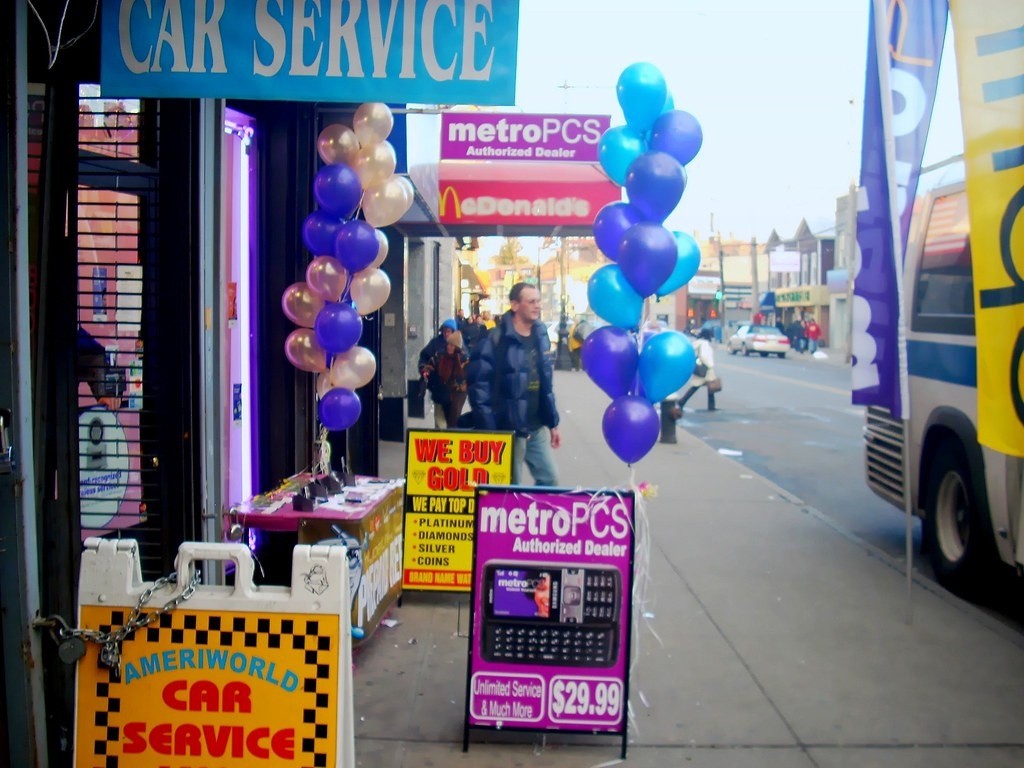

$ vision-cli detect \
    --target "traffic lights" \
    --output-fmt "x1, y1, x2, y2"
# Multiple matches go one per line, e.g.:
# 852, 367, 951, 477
715, 291, 722, 308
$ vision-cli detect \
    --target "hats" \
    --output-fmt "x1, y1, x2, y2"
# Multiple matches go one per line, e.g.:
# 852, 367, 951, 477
441, 319, 456, 331
446, 331, 462, 349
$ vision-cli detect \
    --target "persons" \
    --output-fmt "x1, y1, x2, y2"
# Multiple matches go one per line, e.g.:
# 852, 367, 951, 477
568, 319, 594, 372
28, 263, 122, 410
466, 281, 561, 485
775, 316, 821, 354
418, 309, 500, 429
678, 328, 723, 411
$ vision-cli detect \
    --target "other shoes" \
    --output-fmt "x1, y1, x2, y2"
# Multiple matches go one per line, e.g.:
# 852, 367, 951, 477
709, 407, 719, 410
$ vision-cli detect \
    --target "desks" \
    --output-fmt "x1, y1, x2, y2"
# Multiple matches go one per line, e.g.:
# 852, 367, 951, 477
228, 470, 406, 652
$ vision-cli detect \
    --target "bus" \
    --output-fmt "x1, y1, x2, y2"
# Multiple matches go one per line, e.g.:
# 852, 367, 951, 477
864, 152, 1024, 603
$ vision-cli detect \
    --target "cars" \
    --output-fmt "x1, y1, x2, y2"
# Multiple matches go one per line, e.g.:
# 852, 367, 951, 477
696, 320, 721, 336
545, 322, 572, 352
728, 324, 790, 357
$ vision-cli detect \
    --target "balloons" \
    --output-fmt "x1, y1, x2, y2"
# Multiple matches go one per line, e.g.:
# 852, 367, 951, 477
580, 61, 703, 467
281, 103, 414, 431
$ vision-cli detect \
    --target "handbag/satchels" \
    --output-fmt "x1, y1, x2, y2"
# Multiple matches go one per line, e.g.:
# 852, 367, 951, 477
692, 358, 707, 377
416, 376, 428, 401
706, 378, 721, 394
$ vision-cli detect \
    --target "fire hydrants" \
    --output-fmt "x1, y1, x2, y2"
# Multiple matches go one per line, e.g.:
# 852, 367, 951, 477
660, 394, 680, 444
